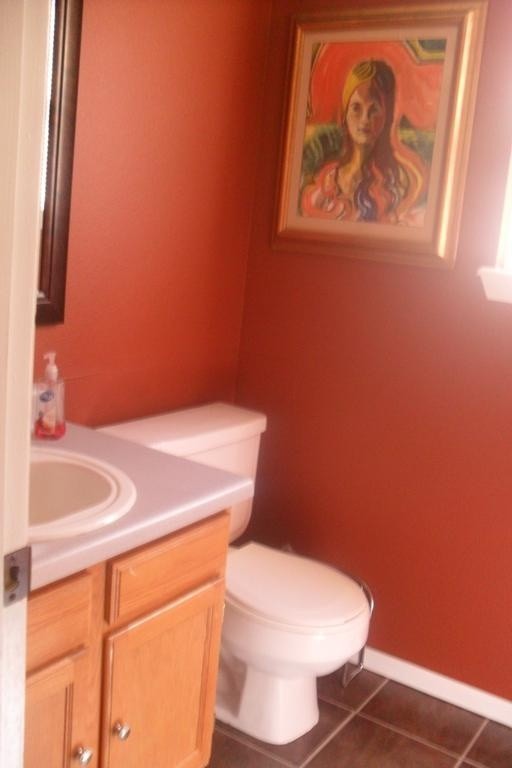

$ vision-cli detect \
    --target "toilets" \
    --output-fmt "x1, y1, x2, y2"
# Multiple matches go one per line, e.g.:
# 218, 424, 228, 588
93, 400, 372, 746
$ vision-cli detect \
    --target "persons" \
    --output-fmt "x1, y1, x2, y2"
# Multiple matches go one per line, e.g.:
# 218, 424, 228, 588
298, 57, 432, 233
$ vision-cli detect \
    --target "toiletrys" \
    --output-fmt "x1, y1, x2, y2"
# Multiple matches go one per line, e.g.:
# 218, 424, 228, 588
34, 352, 66, 439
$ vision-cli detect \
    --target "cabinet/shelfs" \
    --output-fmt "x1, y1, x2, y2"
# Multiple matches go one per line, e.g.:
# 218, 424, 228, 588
23, 508, 231, 767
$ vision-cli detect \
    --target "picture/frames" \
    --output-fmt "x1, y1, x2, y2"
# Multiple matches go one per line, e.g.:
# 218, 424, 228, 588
32, 0, 85, 325
266, 1, 490, 271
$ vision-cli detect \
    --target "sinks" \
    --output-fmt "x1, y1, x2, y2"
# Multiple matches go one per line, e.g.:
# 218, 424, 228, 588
27, 444, 138, 543
